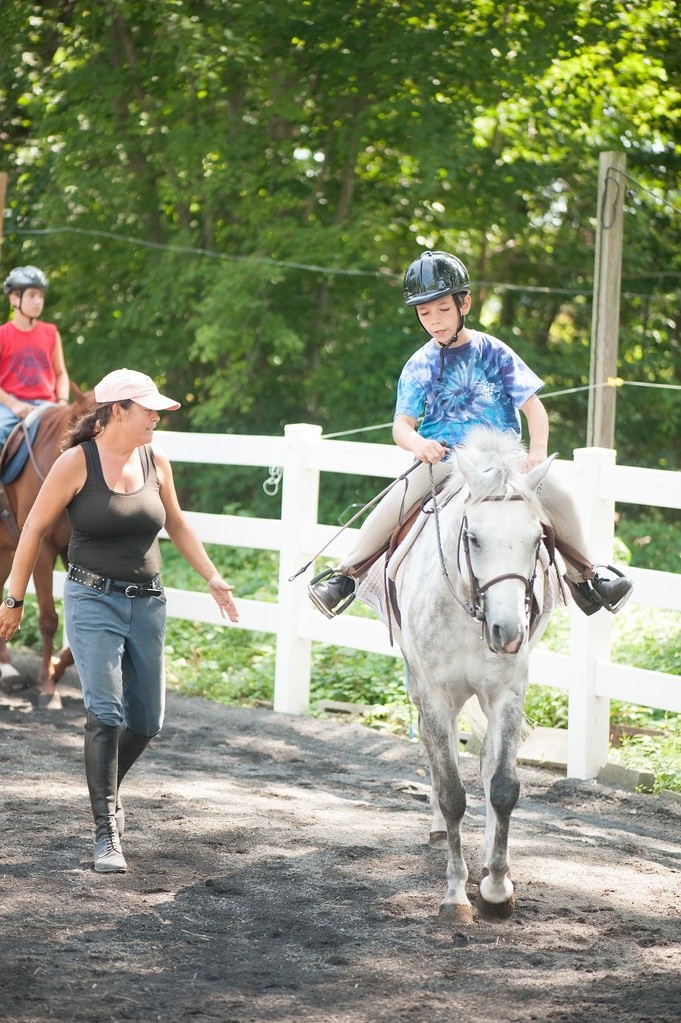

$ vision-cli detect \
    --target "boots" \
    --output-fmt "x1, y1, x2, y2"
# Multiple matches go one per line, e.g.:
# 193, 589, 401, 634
115, 724, 159, 837
83, 710, 126, 872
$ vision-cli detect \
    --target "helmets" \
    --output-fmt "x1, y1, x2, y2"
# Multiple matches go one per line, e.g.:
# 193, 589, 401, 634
402, 250, 470, 305
2, 265, 47, 290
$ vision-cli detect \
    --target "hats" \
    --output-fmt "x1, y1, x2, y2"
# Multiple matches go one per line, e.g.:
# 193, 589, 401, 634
93, 368, 180, 411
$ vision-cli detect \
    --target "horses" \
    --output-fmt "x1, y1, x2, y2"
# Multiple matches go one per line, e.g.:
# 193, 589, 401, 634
383, 424, 565, 925
0, 379, 104, 710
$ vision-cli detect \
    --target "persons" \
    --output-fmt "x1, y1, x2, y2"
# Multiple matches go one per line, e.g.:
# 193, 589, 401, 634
0, 367, 240, 874
0, 265, 71, 462
315, 250, 634, 613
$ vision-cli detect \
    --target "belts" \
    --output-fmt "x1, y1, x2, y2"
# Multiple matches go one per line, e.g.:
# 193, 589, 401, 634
67, 563, 162, 598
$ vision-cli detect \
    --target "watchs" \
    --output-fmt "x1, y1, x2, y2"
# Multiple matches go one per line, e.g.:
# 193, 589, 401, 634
3, 595, 25, 608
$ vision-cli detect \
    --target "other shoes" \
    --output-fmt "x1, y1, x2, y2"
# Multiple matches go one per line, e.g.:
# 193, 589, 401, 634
562, 573, 633, 616
307, 576, 362, 614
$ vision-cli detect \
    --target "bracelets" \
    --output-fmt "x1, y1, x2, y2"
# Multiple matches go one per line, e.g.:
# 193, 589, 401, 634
57, 397, 69, 403
